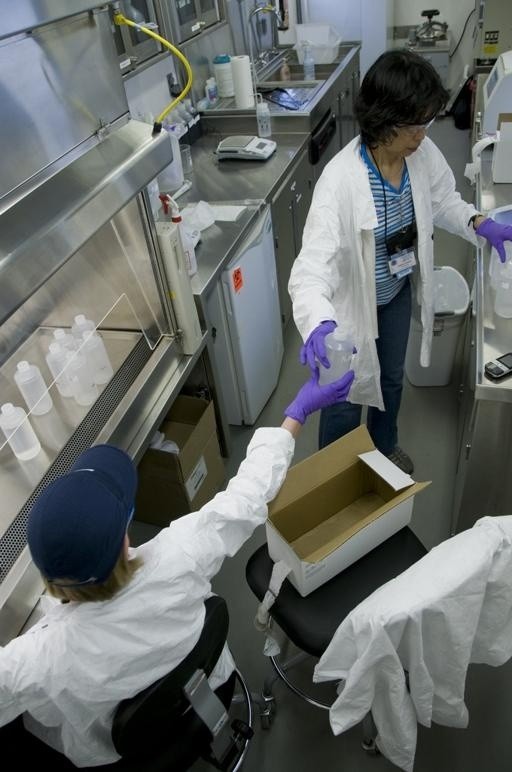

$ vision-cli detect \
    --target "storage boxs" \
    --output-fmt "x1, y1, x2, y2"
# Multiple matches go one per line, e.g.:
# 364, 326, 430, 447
259, 423, 432, 598
136, 395, 235, 534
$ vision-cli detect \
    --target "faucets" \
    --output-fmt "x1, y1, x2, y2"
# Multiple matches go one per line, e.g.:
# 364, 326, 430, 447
249, 6, 285, 58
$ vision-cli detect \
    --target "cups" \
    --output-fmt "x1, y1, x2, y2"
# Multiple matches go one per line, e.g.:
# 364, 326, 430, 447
179, 144, 193, 174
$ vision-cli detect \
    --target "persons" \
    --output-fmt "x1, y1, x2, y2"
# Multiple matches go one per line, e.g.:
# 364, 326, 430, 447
287, 50, 512, 473
0, 368, 356, 771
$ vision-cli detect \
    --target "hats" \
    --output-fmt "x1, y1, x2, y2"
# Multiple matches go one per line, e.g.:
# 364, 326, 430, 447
27, 445, 137, 588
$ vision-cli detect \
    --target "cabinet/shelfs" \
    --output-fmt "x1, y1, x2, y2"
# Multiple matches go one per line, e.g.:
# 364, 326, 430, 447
271, 150, 323, 334
309, 67, 362, 180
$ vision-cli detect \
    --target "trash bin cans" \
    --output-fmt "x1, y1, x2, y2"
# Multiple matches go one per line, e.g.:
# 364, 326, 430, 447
403, 265, 470, 386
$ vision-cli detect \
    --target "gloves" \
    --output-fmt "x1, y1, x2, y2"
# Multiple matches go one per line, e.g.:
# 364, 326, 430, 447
284, 367, 353, 428
299, 323, 357, 371
474, 217, 512, 264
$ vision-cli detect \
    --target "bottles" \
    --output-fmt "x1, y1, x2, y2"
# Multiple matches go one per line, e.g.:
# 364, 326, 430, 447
45, 314, 114, 406
256, 102, 272, 137
302, 45, 315, 81
14, 361, 53, 416
318, 324, 354, 388
490, 240, 512, 319
0, 403, 41, 460
163, 99, 199, 136
212, 54, 235, 100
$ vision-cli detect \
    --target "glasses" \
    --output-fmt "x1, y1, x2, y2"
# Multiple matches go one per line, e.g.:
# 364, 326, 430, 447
394, 119, 436, 135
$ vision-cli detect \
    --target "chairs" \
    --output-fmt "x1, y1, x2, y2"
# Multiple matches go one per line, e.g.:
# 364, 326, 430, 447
1, 590, 273, 769
246, 517, 512, 757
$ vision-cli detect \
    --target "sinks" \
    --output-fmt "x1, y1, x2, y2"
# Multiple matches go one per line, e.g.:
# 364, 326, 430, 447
261, 63, 339, 82
288, 46, 352, 63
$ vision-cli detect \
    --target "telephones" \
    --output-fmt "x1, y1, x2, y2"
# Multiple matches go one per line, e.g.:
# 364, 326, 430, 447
215, 135, 278, 160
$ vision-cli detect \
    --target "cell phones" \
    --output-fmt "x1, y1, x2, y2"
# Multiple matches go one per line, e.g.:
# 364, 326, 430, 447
484, 351, 512, 380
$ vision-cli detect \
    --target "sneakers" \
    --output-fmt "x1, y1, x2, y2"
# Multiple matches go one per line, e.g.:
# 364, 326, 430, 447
388, 446, 414, 475
